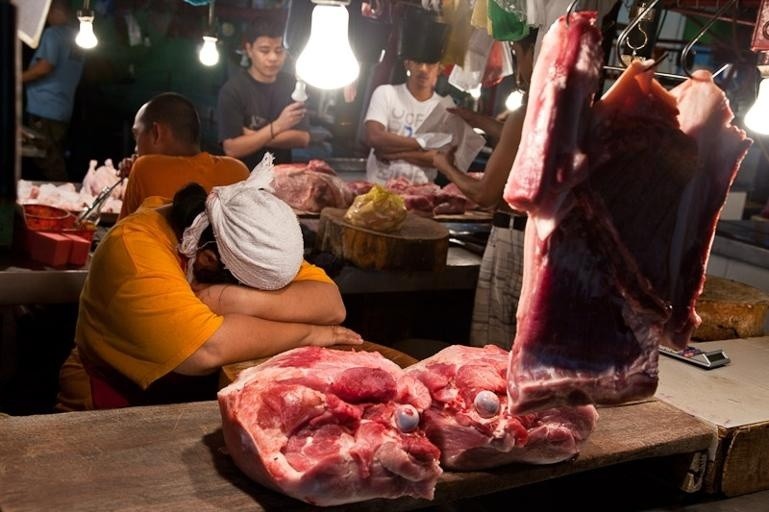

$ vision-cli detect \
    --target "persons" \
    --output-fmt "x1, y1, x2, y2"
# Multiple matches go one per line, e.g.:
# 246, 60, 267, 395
21, 1, 88, 182
219, 14, 312, 174
431, 21, 539, 349
117, 92, 250, 224
55, 148, 364, 414
364, 13, 463, 188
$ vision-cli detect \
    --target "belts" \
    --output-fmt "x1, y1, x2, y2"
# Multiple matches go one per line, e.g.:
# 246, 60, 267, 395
492, 211, 526, 231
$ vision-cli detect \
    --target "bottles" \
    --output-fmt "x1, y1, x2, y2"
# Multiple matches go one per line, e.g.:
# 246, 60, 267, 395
74, 185, 111, 231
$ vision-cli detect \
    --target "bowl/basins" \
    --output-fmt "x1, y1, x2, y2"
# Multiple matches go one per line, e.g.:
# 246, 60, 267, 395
21, 202, 71, 229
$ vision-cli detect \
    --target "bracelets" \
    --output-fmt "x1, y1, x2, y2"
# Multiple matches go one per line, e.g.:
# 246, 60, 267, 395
269, 121, 275, 142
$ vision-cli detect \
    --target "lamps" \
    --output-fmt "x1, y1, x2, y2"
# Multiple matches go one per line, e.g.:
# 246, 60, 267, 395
73, 0, 99, 51
199, 0, 223, 67
293, 0, 362, 89
290, 72, 309, 102
744, 49, 769, 138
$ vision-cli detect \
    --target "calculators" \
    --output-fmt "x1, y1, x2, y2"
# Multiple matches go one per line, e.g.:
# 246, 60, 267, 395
657, 343, 731, 370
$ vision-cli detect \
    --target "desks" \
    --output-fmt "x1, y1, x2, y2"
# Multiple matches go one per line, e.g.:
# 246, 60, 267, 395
652, 332, 769, 512
0, 360, 718, 512
291, 215, 482, 311
